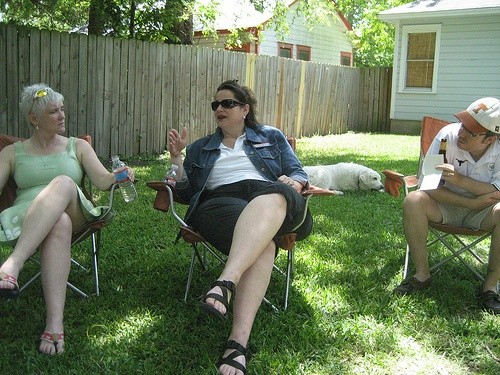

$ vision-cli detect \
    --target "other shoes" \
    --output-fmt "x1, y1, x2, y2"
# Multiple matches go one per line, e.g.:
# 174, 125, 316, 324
479, 285, 500, 315
392, 276, 433, 295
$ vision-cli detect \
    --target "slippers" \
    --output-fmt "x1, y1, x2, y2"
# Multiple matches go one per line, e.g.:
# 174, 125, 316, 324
0, 269, 20, 299
38, 330, 65, 356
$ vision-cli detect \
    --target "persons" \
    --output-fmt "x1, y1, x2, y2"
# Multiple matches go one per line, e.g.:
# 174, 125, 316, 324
169, 79, 310, 375
0, 83, 135, 354
394, 97, 500, 314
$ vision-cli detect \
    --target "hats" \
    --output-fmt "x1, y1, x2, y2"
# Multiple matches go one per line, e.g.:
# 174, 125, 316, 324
452, 96, 500, 136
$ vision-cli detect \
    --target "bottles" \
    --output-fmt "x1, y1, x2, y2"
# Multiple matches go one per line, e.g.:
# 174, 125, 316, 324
111, 155, 138, 202
161, 164, 179, 187
438, 138, 448, 186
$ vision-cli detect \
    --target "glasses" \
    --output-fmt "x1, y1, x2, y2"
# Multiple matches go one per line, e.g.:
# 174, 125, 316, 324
27, 87, 54, 114
211, 98, 245, 111
460, 123, 487, 138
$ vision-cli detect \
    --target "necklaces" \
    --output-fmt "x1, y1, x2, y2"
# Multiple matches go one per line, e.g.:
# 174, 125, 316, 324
30, 138, 57, 168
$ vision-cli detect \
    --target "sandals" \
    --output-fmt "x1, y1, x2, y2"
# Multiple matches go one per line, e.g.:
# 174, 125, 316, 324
218, 338, 247, 375
199, 280, 237, 322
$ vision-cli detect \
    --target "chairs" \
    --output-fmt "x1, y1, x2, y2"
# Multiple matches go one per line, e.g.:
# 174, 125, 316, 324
0, 134, 138, 307
383, 116, 500, 297
145, 136, 344, 318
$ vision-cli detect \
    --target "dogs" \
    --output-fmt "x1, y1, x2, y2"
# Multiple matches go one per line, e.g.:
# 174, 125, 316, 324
302, 163, 384, 195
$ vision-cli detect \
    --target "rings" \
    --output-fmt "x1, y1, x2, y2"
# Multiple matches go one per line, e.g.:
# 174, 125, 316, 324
448, 173, 450, 177
